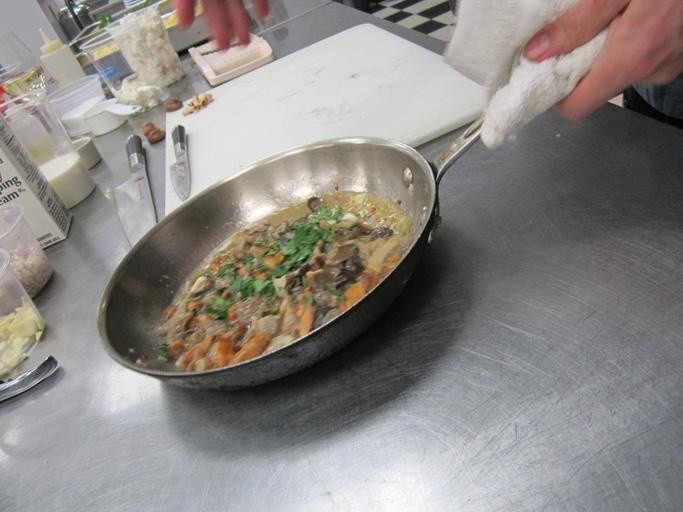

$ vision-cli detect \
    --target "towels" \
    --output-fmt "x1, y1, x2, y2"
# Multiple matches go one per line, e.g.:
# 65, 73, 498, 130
436, 0, 613, 153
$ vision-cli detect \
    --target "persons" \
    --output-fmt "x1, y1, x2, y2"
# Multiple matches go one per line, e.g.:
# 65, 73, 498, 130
167, 0, 683, 124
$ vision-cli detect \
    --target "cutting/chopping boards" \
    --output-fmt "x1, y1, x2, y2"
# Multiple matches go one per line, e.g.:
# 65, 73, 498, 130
164, 21, 487, 217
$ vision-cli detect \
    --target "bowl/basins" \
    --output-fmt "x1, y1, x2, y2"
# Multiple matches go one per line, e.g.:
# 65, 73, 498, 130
0, 203, 54, 299
0, 247, 46, 380
47, 74, 106, 138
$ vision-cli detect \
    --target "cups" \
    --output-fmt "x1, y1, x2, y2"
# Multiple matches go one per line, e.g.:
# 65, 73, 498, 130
105, 7, 186, 88
0, 90, 97, 211
79, 21, 171, 116
55, 137, 102, 172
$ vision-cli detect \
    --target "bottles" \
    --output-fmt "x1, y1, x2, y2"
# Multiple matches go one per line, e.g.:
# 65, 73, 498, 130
38, 27, 86, 88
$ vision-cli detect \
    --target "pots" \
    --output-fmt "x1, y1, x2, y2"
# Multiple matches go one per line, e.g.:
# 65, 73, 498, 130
96, 112, 483, 390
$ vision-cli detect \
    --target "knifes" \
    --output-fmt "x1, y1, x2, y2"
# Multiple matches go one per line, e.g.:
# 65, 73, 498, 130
112, 134, 159, 250
168, 124, 192, 200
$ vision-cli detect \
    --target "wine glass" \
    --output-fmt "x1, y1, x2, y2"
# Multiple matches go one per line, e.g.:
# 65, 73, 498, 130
0, 29, 70, 143
